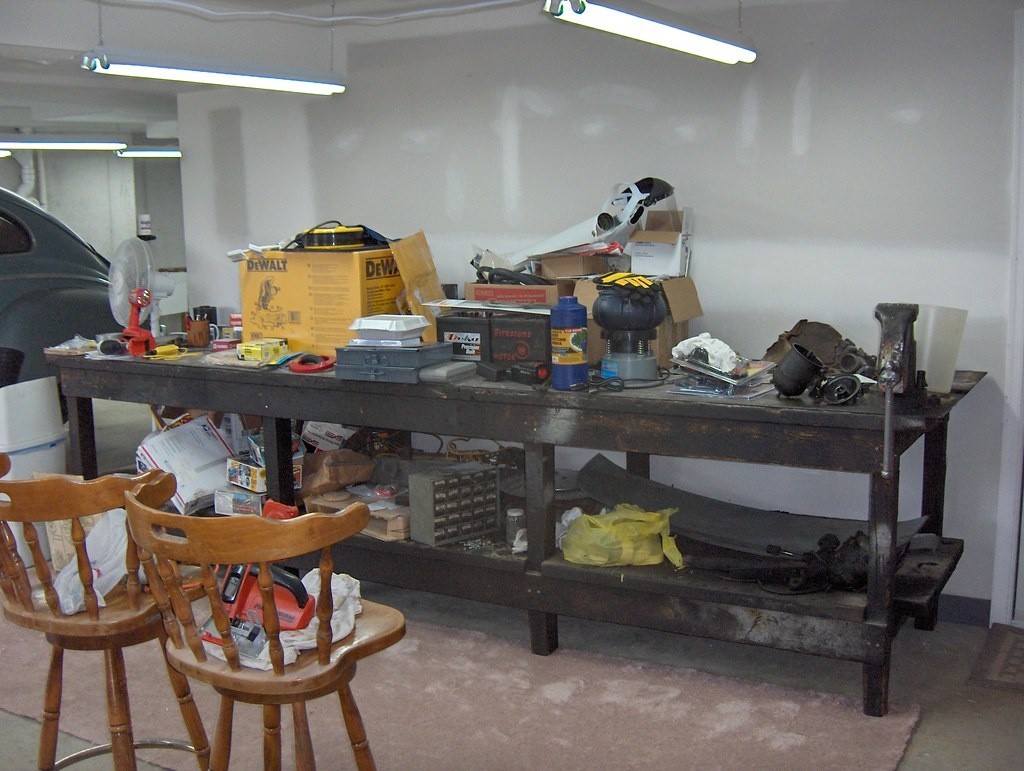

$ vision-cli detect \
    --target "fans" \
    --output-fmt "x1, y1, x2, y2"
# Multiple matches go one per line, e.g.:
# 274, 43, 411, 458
108, 238, 174, 344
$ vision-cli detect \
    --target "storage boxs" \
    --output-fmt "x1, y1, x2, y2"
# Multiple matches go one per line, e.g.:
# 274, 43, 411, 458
465, 278, 573, 305
572, 278, 702, 372
214, 419, 364, 515
489, 314, 553, 365
543, 252, 606, 277
434, 316, 491, 363
239, 246, 403, 356
622, 210, 689, 277
134, 415, 242, 517
332, 342, 453, 384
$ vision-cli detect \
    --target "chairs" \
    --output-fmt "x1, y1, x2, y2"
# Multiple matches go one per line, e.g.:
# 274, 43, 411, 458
125, 471, 406, 771
0, 453, 216, 771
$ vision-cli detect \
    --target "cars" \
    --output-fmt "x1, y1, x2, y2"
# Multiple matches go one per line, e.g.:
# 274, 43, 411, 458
0, 188, 121, 418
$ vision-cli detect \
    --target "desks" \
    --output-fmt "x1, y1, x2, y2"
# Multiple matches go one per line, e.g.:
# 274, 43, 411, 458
42, 305, 991, 721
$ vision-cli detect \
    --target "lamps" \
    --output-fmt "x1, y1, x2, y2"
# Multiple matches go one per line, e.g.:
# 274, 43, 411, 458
0, 133, 183, 158
544, 0, 758, 65
81, 0, 346, 96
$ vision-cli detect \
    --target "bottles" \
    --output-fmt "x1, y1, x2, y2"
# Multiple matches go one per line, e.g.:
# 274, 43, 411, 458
506, 508, 526, 544
551, 296, 588, 390
137, 214, 152, 236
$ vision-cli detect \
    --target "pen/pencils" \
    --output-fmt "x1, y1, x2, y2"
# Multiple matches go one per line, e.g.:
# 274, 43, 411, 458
186, 307, 208, 322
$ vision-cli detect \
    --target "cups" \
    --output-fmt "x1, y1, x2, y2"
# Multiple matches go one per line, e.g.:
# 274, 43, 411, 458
187, 320, 219, 346
915, 303, 967, 394
